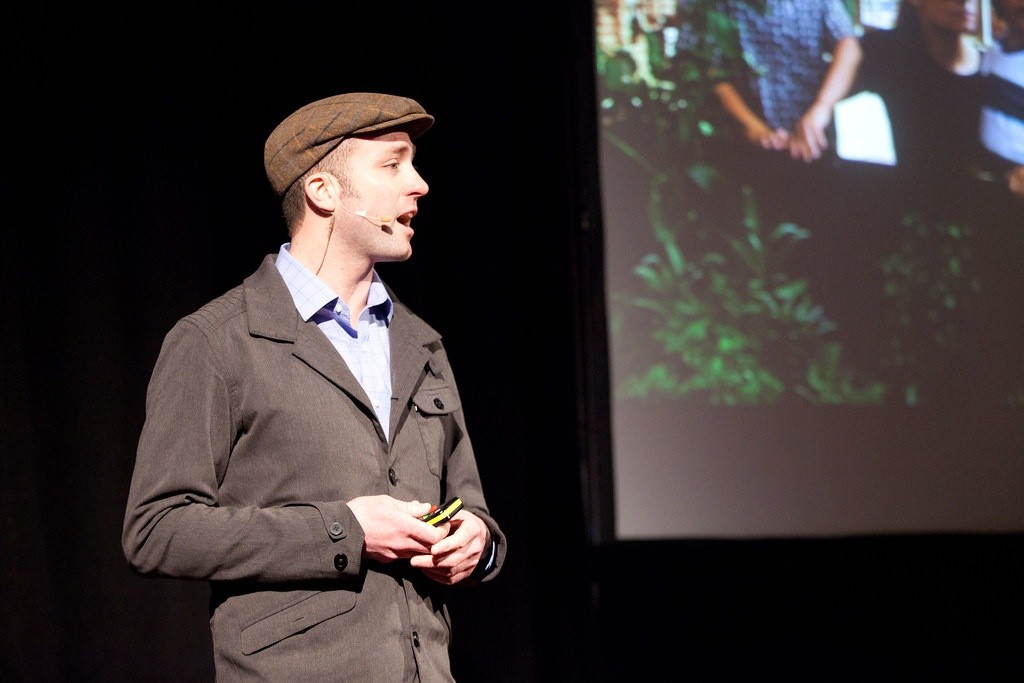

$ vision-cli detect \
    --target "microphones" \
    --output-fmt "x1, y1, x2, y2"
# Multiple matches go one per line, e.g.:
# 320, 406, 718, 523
335, 190, 392, 224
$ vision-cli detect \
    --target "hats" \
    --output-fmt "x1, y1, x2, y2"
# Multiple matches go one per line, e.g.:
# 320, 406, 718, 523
264, 93, 434, 194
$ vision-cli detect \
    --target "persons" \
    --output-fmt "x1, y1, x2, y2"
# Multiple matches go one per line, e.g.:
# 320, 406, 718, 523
122, 93, 507, 683
675, 0, 1024, 204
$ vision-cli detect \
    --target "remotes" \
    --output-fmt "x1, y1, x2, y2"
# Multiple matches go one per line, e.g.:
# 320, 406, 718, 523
417, 497, 463, 528
